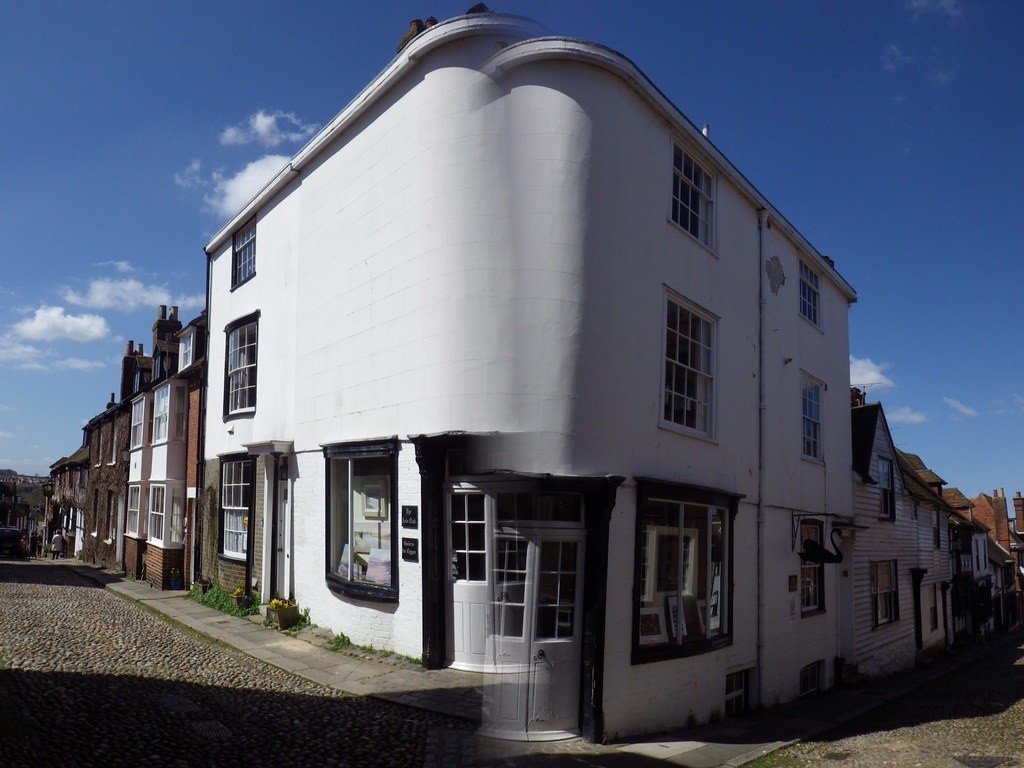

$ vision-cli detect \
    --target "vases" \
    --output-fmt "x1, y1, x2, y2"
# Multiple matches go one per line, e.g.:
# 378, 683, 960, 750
230, 595, 250, 608
197, 580, 212, 595
266, 605, 298, 629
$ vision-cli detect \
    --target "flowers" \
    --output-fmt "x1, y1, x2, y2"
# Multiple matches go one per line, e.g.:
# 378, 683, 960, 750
268, 597, 292, 610
232, 588, 248, 596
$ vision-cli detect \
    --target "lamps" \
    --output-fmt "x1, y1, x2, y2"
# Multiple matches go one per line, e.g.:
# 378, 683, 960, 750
121, 447, 131, 470
228, 425, 234, 435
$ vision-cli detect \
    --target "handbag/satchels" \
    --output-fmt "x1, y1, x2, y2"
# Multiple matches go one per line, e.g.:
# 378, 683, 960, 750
61, 536, 67, 546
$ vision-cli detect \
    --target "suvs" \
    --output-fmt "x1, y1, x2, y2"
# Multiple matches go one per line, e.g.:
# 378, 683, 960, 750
0, 526, 26, 558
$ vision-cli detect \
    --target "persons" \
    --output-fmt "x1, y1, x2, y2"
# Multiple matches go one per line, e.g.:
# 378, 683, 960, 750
51, 530, 63, 559
22, 530, 43, 557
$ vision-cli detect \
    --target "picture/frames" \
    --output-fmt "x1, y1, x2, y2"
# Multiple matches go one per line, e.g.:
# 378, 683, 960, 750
639, 607, 668, 645
361, 478, 387, 517
665, 595, 688, 638
695, 598, 707, 635
